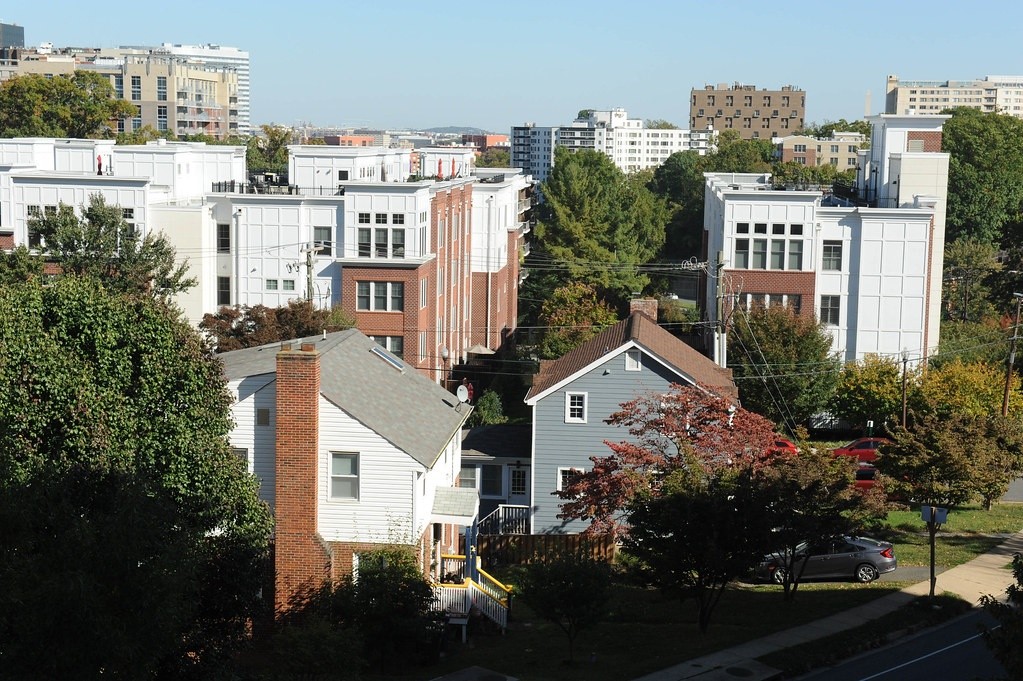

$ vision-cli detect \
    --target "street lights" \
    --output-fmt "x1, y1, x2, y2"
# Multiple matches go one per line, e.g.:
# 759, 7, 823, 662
900, 346, 911, 429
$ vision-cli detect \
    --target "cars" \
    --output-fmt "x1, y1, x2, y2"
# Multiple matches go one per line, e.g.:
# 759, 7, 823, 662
853, 466, 879, 493
833, 437, 896, 459
746, 535, 897, 585
